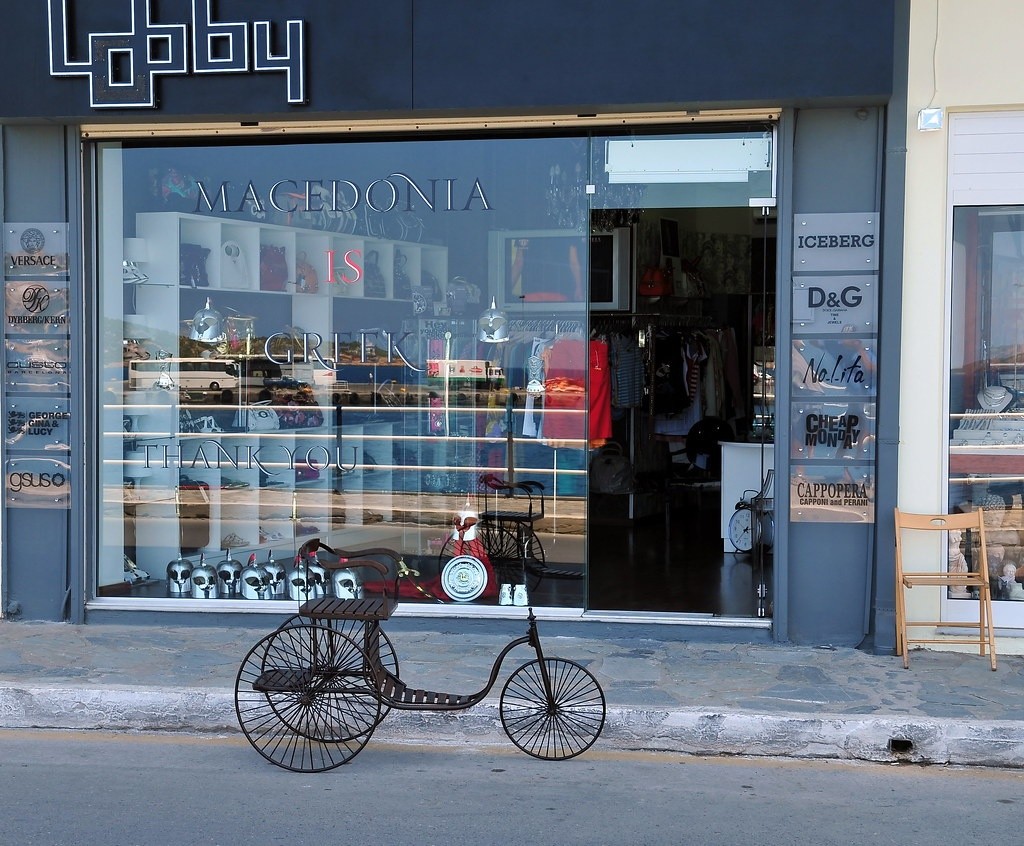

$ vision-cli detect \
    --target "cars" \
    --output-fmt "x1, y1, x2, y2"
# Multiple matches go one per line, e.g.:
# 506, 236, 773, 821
5, 357, 70, 391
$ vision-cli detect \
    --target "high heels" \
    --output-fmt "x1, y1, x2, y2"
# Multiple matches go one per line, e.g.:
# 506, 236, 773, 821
186, 409, 226, 433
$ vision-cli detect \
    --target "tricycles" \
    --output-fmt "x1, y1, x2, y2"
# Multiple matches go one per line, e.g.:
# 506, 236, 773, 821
234, 538, 606, 773
438, 474, 586, 601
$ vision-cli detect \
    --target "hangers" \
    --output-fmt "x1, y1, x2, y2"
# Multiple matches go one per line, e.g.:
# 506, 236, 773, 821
505, 316, 727, 339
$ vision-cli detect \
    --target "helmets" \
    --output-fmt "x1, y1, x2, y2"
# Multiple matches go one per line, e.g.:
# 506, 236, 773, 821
165, 548, 365, 601
475, 295, 509, 343
453, 494, 481, 541
187, 297, 224, 343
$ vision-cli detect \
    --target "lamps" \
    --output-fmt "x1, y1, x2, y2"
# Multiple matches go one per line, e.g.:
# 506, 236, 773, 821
124, 390, 148, 432
123, 314, 150, 340
123, 451, 152, 489
124, 237, 148, 263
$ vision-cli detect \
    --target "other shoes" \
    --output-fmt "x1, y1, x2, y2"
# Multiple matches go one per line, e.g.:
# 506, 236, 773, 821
122, 262, 148, 284
125, 346, 151, 365
221, 477, 249, 490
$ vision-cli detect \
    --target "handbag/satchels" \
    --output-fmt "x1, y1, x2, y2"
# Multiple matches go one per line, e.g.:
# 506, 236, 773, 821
179, 242, 210, 287
394, 250, 413, 299
260, 244, 289, 292
269, 376, 324, 429
231, 400, 280, 431
220, 241, 249, 289
363, 449, 377, 474
588, 441, 637, 494
295, 250, 319, 294
296, 458, 319, 480
364, 249, 386, 298
420, 271, 441, 302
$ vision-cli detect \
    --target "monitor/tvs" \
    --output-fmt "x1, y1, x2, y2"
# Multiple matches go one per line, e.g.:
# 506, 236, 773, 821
487, 226, 631, 312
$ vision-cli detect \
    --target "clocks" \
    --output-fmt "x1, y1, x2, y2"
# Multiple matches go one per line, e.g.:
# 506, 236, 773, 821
727, 487, 775, 559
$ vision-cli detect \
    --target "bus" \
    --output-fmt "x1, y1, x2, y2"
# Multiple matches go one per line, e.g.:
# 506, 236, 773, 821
216, 355, 314, 389
128, 358, 241, 392
280, 358, 336, 386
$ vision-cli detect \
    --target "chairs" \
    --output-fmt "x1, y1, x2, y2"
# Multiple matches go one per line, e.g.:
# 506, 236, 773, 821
894, 509, 998, 672
476, 473, 544, 522
296, 538, 404, 621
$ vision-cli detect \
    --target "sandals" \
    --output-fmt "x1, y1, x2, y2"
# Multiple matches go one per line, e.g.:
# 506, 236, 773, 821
257, 527, 284, 542
221, 532, 249, 550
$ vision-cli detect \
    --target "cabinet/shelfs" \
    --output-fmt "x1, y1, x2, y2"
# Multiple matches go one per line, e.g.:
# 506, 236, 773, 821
718, 441, 774, 554
133, 210, 450, 579
123, 282, 176, 507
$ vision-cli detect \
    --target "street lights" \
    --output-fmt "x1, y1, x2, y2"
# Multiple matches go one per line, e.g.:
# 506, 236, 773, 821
184, 316, 257, 356
444, 331, 451, 437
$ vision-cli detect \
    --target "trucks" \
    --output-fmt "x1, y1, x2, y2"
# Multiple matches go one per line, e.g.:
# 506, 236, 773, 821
427, 359, 506, 390
